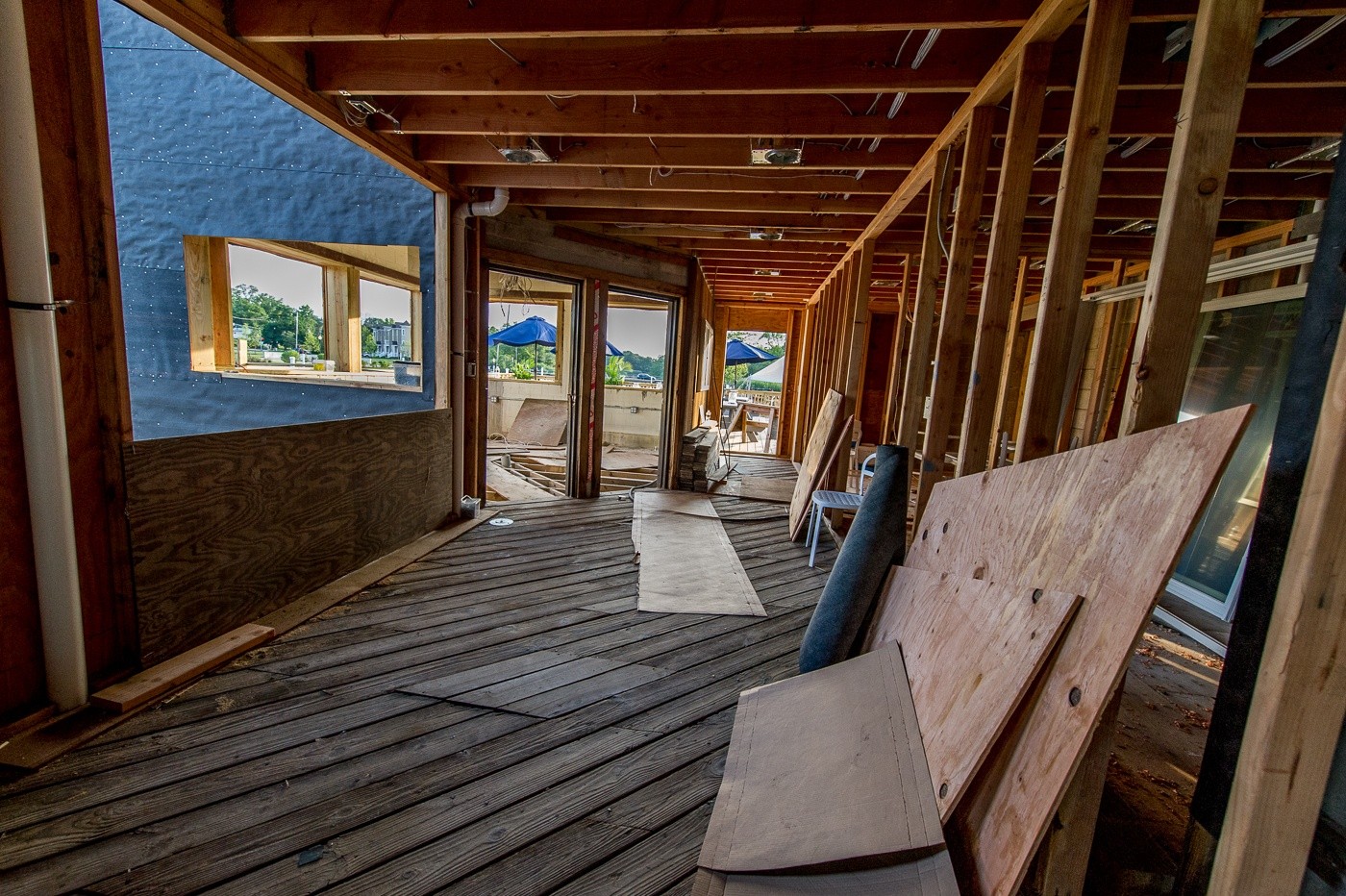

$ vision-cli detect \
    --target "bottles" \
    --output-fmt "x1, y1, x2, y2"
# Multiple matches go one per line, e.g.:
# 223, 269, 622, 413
706, 410, 711, 422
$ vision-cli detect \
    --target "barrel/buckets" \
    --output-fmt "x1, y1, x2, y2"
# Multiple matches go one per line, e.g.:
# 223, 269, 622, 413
501, 454, 511, 467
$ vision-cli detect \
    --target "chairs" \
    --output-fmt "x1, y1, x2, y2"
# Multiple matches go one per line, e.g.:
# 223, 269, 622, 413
805, 453, 876, 569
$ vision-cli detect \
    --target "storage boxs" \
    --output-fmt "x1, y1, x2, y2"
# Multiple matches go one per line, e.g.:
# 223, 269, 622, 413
393, 361, 421, 386
312, 360, 335, 372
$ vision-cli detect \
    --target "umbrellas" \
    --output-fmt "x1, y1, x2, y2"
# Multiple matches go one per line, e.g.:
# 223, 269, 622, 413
726, 337, 780, 390
489, 316, 557, 379
746, 356, 785, 384
550, 339, 625, 356
